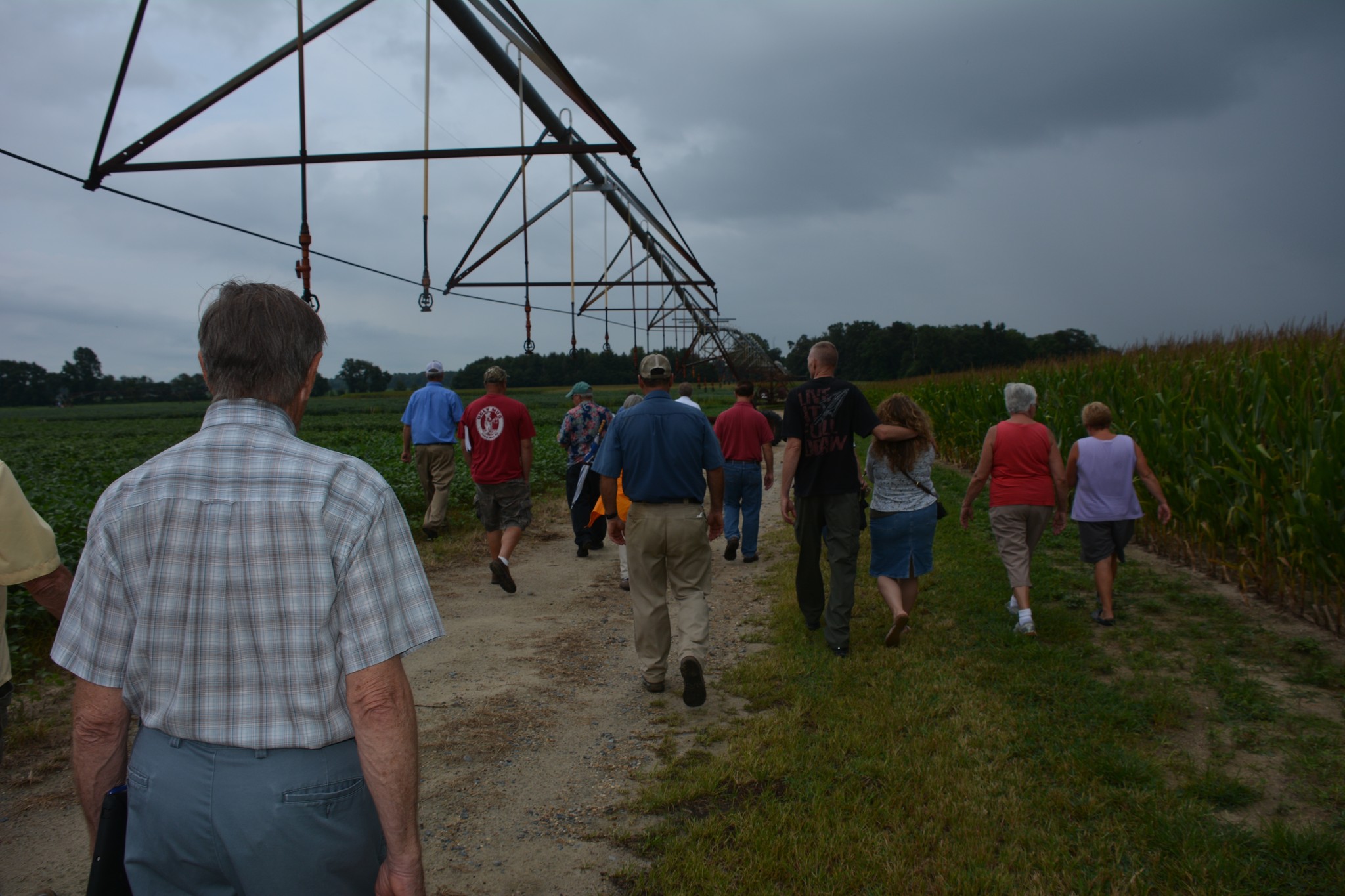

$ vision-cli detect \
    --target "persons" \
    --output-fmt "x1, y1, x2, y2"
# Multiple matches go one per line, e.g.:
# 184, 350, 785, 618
853, 441, 868, 497
960, 383, 1068, 636
557, 381, 614, 557
588, 393, 644, 592
866, 393, 938, 648
781, 340, 922, 656
457, 366, 537, 593
1065, 402, 1171, 626
400, 360, 465, 536
0, 457, 73, 733
616, 392, 636, 414
50, 279, 445, 896
713, 379, 775, 563
674, 381, 702, 410
591, 354, 726, 707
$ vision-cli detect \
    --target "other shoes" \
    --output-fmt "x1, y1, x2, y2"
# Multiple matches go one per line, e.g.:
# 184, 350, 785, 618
1018, 620, 1037, 643
724, 537, 739, 561
1010, 606, 1019, 618
589, 541, 604, 550
832, 648, 849, 654
743, 555, 758, 563
577, 540, 589, 557
1096, 596, 1103, 606
680, 656, 706, 707
489, 557, 516, 594
645, 679, 666, 692
806, 622, 820, 630
885, 611, 908, 647
1091, 609, 1116, 626
619, 579, 630, 590
491, 574, 499, 584
422, 525, 438, 539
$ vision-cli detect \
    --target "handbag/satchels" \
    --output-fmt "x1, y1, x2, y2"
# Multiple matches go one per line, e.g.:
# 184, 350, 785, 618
859, 486, 870, 530
937, 501, 948, 520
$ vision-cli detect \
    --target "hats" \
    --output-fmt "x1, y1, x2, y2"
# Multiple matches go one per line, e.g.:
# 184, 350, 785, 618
639, 353, 672, 379
483, 365, 510, 384
565, 382, 593, 397
426, 360, 443, 374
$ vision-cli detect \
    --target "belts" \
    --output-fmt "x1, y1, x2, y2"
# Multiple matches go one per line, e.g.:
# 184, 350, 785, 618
726, 459, 760, 464
632, 499, 698, 504
